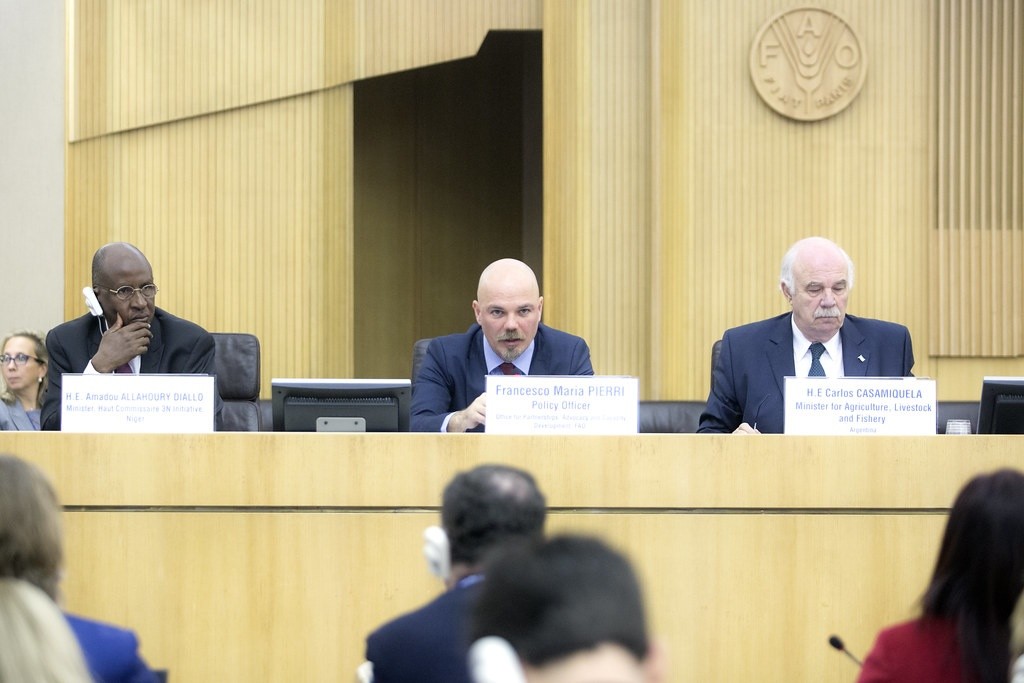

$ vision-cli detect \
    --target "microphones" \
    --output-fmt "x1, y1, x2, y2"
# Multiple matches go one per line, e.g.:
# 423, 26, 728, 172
830, 636, 862, 666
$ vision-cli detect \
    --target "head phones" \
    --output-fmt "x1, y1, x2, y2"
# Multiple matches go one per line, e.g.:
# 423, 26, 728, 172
423, 525, 451, 580
466, 634, 525, 683
83, 287, 103, 317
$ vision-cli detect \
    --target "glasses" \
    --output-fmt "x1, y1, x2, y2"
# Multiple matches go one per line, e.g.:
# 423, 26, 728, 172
93, 284, 159, 300
0, 354, 45, 366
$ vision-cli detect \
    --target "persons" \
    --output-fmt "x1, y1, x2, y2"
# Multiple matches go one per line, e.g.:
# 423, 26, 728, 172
0, 452, 166, 683
0, 328, 54, 431
361, 463, 548, 683
854, 466, 1024, 683
695, 236, 915, 433
404, 256, 594, 432
40, 242, 226, 431
468, 532, 666, 682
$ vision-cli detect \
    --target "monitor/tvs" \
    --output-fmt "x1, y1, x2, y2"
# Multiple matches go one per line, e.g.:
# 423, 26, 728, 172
975, 377, 1024, 434
271, 378, 411, 432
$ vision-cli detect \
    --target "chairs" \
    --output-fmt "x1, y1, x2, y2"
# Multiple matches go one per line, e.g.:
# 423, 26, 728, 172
204, 331, 981, 434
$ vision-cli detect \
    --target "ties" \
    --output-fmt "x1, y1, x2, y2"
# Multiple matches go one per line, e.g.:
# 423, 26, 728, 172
498, 362, 514, 375
114, 362, 133, 373
808, 343, 826, 376
